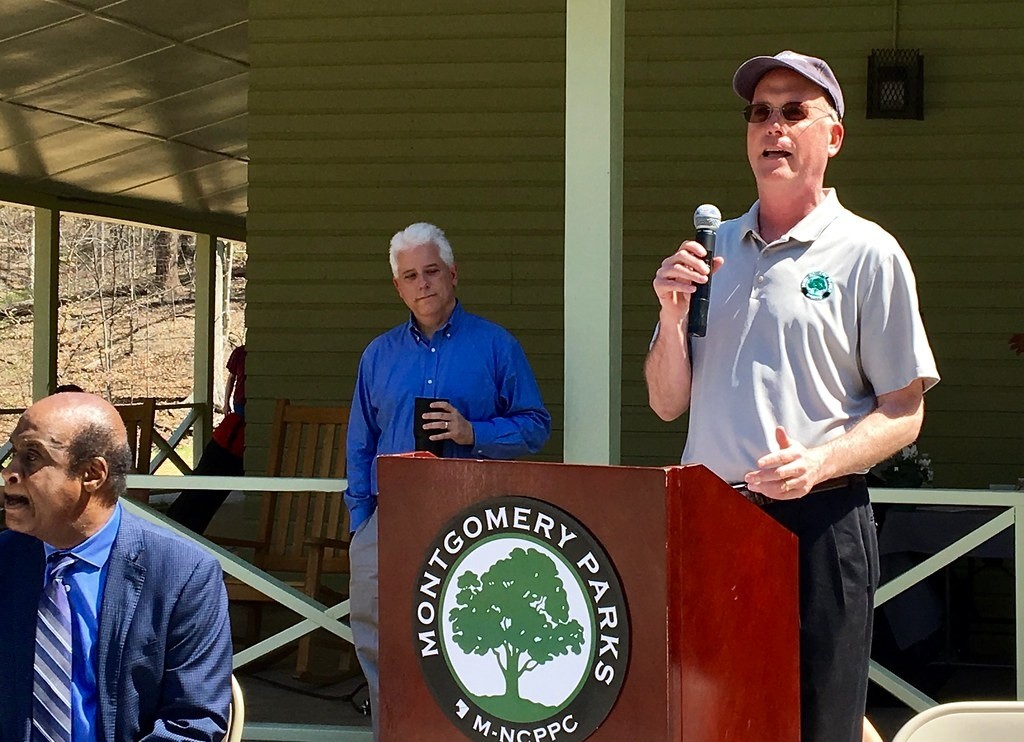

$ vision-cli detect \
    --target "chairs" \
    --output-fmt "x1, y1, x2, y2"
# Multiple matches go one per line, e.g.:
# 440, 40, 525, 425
114, 398, 153, 504
196, 399, 361, 686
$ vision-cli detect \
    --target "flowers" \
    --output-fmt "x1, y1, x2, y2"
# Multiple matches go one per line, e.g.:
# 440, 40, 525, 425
881, 443, 935, 484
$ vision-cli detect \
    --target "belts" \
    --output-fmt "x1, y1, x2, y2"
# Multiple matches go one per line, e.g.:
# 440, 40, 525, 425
731, 474, 866, 506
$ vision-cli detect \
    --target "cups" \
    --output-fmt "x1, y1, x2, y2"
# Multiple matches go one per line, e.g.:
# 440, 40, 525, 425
414, 397, 450, 457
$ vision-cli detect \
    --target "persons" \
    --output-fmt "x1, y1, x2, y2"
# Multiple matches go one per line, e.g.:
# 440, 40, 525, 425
643, 51, 941, 742
0, 390, 234, 742
346, 219, 553, 741
224, 345, 247, 417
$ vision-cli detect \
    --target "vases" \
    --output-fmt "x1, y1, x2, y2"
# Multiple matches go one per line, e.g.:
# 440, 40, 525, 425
886, 479, 923, 512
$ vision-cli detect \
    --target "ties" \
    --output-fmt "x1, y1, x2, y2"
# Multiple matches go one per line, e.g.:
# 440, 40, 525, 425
31, 553, 80, 742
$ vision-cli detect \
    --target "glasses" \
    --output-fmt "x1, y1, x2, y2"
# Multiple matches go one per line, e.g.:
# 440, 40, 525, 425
740, 102, 836, 124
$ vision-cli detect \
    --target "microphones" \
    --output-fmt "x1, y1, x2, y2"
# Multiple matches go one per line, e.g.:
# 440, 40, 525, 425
688, 203, 722, 337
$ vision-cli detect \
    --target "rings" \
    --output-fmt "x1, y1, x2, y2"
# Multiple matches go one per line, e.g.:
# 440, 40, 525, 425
445, 422, 448, 428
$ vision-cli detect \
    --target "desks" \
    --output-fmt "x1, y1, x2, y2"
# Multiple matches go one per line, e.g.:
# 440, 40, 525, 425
870, 504, 1015, 674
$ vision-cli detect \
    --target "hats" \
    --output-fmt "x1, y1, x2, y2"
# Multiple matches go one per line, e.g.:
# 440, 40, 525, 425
733, 50, 844, 124
